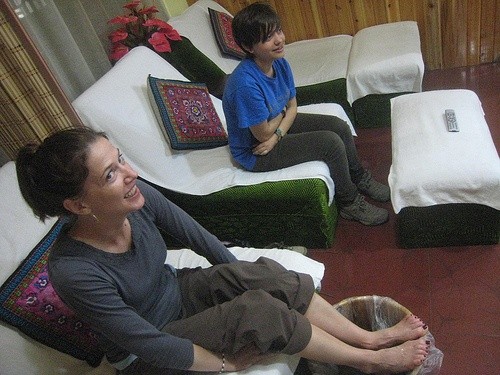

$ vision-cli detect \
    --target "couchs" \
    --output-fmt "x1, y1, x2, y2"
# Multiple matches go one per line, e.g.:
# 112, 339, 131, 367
71, 46, 359, 251
0, 160, 325, 375
160, 0, 352, 113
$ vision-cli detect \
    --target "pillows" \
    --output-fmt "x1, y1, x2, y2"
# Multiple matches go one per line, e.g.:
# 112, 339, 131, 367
208, 8, 250, 60
0, 219, 103, 368
149, 76, 228, 152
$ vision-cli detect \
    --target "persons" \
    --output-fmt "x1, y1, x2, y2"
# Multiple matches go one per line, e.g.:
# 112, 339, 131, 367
222, 1, 390, 225
16, 127, 430, 375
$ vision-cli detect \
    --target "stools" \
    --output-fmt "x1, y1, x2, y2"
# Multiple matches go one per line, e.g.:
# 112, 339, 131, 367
346, 21, 427, 125
385, 85, 500, 247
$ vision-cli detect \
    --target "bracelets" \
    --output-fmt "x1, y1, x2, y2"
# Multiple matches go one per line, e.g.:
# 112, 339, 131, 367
217, 352, 225, 373
275, 128, 283, 141
281, 110, 286, 118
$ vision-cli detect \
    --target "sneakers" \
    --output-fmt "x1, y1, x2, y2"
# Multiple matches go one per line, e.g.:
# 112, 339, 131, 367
340, 194, 388, 226
366, 181, 390, 202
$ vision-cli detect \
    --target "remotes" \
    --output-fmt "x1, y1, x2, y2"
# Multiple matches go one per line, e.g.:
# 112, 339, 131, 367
445, 109, 459, 131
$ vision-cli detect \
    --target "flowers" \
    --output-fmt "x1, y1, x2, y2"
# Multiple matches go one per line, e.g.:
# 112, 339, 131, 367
108, 1, 179, 63
